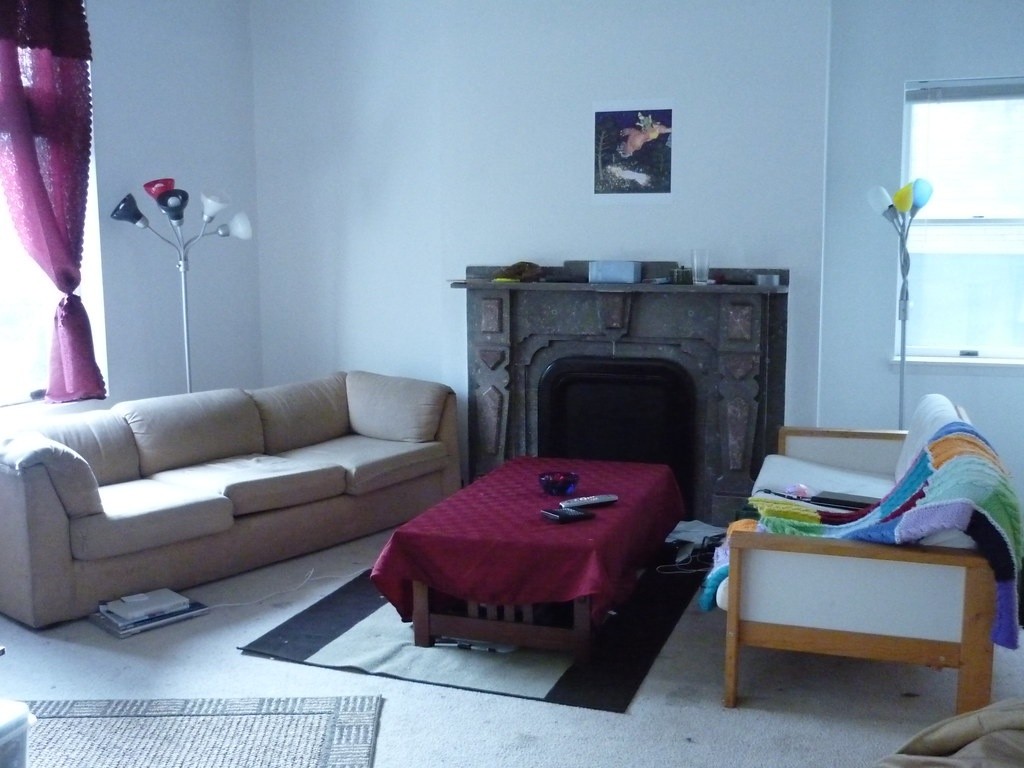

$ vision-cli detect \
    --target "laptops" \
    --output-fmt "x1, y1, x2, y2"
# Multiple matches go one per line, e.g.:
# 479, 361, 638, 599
90, 598, 209, 640
810, 491, 881, 512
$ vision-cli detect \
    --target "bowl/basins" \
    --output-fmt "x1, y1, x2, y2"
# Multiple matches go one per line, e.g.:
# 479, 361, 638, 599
538, 470, 579, 496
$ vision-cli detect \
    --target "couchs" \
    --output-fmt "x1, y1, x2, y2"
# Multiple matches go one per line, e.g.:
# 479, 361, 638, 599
0, 368, 462, 631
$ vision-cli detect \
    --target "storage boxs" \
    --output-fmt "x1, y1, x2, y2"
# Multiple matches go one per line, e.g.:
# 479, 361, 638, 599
588, 261, 642, 284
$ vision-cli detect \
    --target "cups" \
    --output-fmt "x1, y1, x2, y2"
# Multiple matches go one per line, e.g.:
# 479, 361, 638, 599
690, 247, 709, 286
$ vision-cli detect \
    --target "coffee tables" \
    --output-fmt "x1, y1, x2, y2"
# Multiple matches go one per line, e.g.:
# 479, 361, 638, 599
394, 456, 671, 666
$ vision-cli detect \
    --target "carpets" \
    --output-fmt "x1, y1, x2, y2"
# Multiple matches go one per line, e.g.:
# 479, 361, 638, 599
19, 693, 383, 768
233, 543, 716, 714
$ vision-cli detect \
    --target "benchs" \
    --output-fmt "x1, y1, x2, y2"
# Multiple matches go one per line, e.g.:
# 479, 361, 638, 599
720, 392, 1016, 717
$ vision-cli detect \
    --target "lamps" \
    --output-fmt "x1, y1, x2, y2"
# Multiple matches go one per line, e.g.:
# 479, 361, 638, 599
109, 177, 251, 392
863, 179, 934, 431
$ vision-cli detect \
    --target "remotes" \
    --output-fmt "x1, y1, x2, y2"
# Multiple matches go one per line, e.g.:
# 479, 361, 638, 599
560, 494, 619, 509
